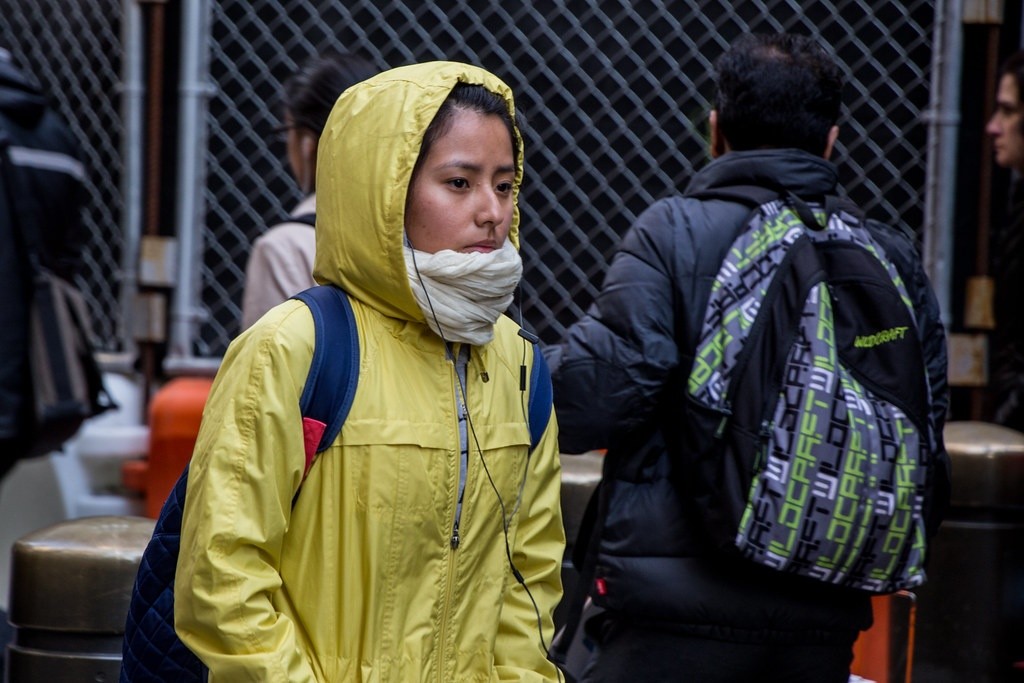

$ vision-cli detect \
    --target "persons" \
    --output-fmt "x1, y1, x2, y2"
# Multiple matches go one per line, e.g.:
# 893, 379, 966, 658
174, 61, 565, 683
542, 35, 949, 683
0, 54, 86, 486
244, 55, 380, 329
980, 50, 1024, 434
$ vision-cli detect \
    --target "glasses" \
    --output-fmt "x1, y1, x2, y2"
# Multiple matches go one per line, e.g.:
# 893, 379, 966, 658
268, 122, 302, 143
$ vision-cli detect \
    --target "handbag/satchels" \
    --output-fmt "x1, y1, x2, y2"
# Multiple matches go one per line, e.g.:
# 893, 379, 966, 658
32, 273, 118, 419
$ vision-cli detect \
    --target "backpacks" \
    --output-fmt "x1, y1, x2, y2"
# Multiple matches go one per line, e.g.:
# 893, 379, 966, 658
122, 284, 552, 683
650, 182, 936, 592
